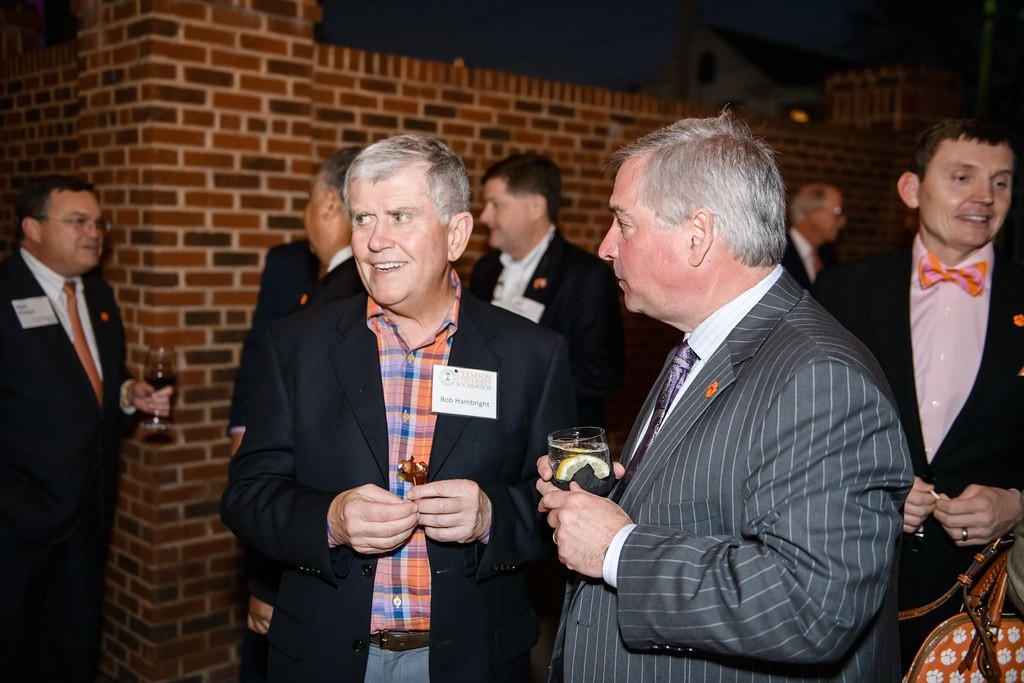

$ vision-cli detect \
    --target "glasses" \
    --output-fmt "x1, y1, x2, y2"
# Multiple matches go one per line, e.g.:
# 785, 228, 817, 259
36, 216, 111, 233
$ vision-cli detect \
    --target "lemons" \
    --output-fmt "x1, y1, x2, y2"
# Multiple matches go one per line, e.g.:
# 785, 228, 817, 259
555, 455, 610, 481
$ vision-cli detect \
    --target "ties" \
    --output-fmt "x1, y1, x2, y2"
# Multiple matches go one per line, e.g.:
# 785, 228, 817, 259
610, 337, 698, 506
63, 281, 102, 407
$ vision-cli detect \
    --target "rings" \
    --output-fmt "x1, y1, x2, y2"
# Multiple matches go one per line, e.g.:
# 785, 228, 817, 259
962, 527, 968, 542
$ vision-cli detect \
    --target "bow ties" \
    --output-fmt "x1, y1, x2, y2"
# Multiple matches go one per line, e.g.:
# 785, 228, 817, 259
918, 251, 987, 298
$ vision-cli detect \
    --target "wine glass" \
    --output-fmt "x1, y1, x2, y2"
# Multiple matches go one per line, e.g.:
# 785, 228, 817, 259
140, 347, 176, 430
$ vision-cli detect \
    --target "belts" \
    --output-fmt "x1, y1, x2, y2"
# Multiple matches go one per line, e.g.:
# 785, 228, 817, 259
369, 629, 430, 652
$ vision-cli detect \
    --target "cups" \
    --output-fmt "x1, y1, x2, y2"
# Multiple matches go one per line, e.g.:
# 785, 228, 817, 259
547, 426, 615, 497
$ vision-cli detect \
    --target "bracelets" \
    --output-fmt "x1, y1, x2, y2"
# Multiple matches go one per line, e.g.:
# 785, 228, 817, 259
122, 380, 136, 408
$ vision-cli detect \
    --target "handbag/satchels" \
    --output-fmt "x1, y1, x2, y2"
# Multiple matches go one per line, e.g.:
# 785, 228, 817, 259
899, 536, 1024, 683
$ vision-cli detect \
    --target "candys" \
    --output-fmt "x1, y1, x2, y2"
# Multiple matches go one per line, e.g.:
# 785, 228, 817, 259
401, 455, 429, 486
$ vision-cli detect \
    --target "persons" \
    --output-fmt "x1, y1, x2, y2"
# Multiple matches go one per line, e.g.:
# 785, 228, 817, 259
246, 147, 365, 633
783, 182, 845, 299
465, 153, 624, 656
224, 240, 322, 463
1005, 523, 1024, 621
0, 175, 172, 682
819, 108, 1024, 682
218, 133, 576, 683
534, 107, 915, 683
